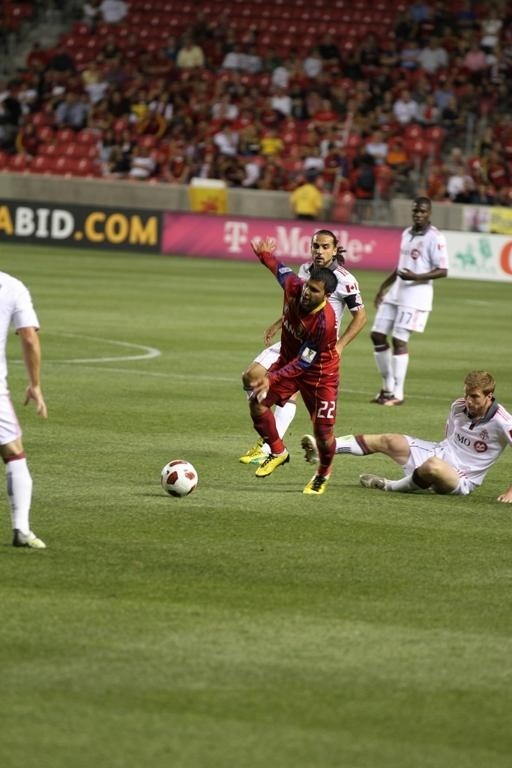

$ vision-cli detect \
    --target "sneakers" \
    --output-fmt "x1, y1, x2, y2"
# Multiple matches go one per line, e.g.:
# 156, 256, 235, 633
358, 473, 395, 492
11, 529, 48, 550
370, 389, 405, 406
299, 434, 320, 465
238, 436, 271, 465
254, 446, 291, 478
301, 469, 332, 496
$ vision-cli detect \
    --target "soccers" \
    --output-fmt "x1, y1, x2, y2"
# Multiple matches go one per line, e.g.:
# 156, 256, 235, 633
160, 460, 198, 497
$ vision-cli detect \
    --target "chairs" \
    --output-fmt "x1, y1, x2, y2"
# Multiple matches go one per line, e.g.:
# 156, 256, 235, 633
0, 0, 512, 208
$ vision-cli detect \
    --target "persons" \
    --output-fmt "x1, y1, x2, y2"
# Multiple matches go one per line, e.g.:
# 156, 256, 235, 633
368, 196, 449, 406
0, 271, 50, 550
237, 230, 367, 465
249, 237, 343, 494
301, 369, 511, 505
